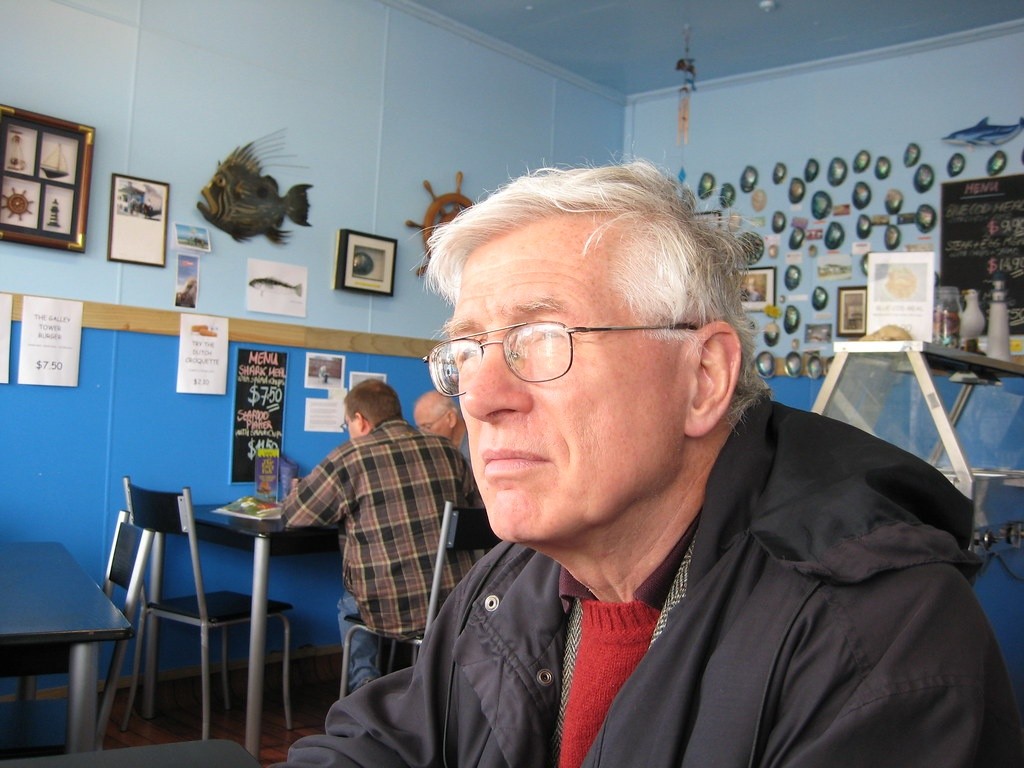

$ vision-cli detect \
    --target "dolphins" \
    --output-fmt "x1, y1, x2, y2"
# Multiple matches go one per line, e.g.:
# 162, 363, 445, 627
941, 116, 1024, 146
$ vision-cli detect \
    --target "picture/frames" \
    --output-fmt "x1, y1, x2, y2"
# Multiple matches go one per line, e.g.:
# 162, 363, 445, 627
838, 286, 867, 337
737, 267, 776, 311
333, 229, 397, 296
0, 103, 96, 253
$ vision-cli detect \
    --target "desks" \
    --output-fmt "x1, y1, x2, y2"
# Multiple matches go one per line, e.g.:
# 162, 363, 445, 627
138, 502, 339, 759
0, 540, 134, 753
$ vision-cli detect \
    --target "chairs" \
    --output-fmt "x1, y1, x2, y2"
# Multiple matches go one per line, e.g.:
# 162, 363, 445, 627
339, 499, 503, 699
10, 478, 296, 747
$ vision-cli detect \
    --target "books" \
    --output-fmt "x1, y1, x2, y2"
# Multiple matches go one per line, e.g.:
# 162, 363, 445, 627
210, 491, 287, 521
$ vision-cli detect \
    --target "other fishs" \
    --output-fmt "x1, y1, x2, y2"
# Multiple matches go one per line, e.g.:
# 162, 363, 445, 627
197, 127, 312, 245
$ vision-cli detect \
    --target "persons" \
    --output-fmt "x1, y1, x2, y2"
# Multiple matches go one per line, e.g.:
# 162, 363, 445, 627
280, 377, 488, 698
284, 164, 1023, 768
410, 387, 474, 470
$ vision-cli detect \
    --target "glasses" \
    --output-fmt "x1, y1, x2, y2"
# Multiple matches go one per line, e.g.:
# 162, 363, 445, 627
418, 410, 449, 433
340, 410, 358, 432
421, 320, 697, 398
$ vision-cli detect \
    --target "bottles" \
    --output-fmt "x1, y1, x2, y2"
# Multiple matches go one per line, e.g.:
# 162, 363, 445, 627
933, 286, 962, 350
986, 270, 1012, 363
